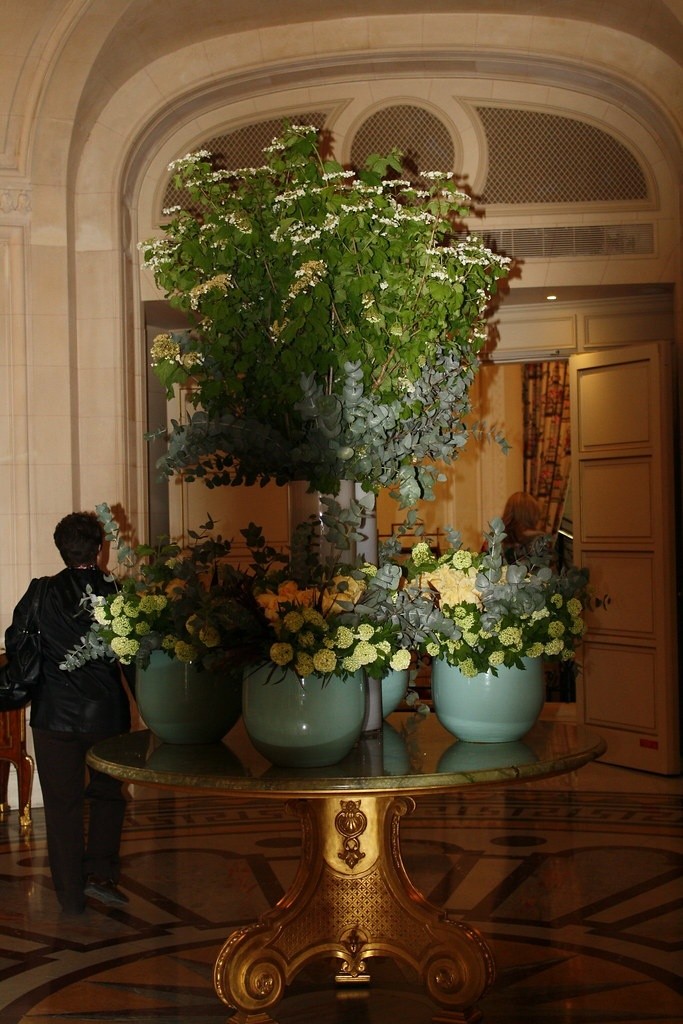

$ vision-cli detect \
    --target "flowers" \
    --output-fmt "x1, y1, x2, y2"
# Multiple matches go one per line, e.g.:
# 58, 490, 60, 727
55, 121, 614, 688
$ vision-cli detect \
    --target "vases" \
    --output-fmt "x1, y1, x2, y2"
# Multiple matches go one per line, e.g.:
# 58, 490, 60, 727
238, 664, 369, 770
283, 479, 386, 736
379, 668, 411, 723
135, 650, 242, 749
428, 651, 549, 746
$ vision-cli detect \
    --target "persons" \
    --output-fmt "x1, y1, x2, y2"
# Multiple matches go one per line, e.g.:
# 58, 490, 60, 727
0, 511, 131, 919
482, 489, 565, 700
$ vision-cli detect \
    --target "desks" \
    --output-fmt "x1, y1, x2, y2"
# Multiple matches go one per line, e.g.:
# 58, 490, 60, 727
85, 712, 608, 1009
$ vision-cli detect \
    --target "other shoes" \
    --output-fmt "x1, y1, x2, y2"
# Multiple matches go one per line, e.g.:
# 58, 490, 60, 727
62, 874, 130, 915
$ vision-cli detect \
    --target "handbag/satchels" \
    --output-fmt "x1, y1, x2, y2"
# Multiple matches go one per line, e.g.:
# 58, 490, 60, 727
0, 630, 43, 711
